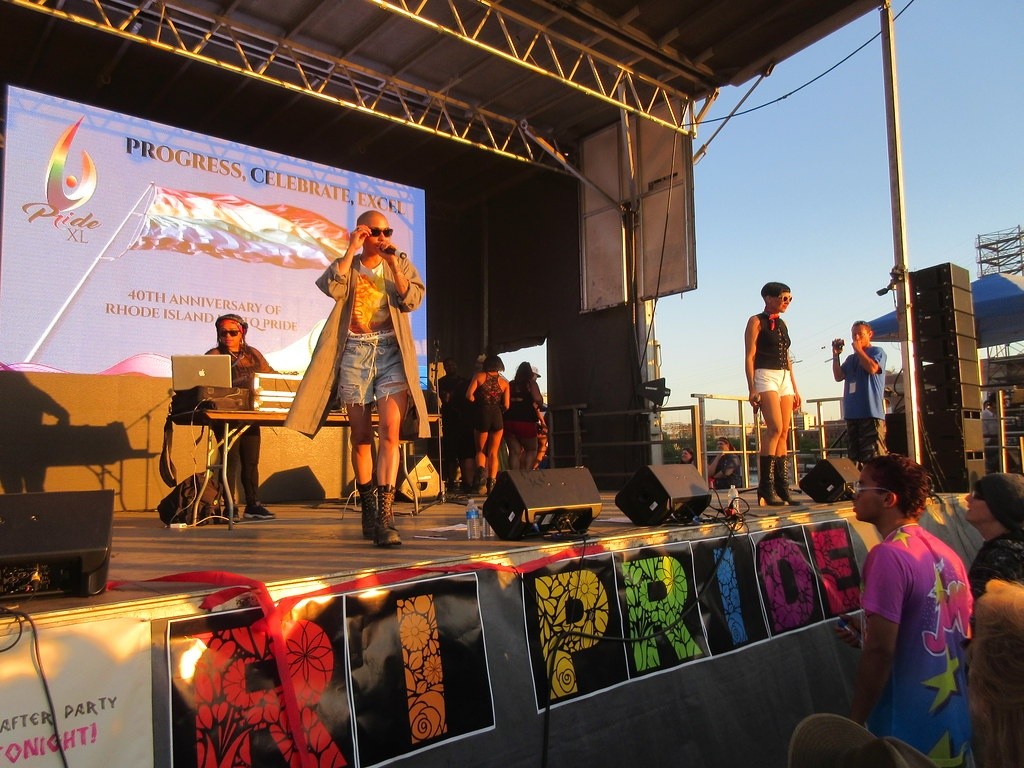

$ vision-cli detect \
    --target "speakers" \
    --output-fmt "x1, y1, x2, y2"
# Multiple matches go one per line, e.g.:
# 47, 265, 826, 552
0, 489, 115, 597
615, 464, 712, 527
909, 262, 986, 493
393, 454, 441, 503
799, 457, 863, 503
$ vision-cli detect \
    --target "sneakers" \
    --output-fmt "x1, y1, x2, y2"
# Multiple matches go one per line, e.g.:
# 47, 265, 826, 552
244, 504, 275, 519
223, 506, 239, 521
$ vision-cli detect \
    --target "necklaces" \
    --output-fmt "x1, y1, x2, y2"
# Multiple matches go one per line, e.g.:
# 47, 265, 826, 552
229, 350, 245, 359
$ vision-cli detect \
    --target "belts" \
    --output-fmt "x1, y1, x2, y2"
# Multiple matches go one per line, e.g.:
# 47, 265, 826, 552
347, 338, 398, 345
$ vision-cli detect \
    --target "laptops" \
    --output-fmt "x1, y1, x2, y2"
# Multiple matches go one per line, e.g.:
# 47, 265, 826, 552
171, 354, 232, 391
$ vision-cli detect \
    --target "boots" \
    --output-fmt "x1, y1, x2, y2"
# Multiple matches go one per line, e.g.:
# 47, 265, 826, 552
774, 455, 800, 505
488, 476, 497, 496
355, 474, 378, 538
373, 484, 402, 545
757, 455, 784, 506
476, 466, 487, 495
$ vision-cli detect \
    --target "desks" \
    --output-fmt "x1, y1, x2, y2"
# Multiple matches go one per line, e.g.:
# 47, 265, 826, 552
168, 410, 442, 530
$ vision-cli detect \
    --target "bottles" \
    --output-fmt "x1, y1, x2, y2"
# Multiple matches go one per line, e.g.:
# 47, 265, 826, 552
465, 499, 480, 540
728, 484, 739, 513
483, 517, 494, 537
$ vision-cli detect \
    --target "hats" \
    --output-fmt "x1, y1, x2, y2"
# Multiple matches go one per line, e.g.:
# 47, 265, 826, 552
789, 713, 939, 768
977, 474, 1024, 530
531, 366, 541, 378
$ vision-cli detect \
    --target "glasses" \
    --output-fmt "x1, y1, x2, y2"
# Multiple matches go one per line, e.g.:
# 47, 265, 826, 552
854, 482, 895, 495
369, 228, 393, 236
769, 295, 792, 302
971, 488, 983, 499
219, 330, 241, 336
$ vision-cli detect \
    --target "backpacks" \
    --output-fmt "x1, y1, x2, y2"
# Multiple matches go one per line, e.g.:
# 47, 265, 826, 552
158, 473, 225, 525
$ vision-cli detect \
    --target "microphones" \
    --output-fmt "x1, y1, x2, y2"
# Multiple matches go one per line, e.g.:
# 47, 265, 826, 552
379, 242, 407, 259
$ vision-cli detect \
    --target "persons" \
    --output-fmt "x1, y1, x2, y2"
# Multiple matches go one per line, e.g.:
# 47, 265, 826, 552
706, 437, 742, 490
679, 447, 695, 464
438, 358, 475, 498
834, 453, 975, 768
204, 314, 283, 522
965, 473, 1024, 636
831, 320, 887, 472
503, 362, 543, 470
981, 392, 1000, 474
787, 578, 1024, 768
316, 211, 431, 546
745, 282, 801, 506
466, 356, 511, 497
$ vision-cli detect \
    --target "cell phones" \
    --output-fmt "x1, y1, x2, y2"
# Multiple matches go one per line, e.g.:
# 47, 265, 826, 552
838, 619, 861, 647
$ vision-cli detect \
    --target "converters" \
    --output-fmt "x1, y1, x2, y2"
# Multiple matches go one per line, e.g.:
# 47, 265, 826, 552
170, 523, 187, 528
482, 467, 602, 541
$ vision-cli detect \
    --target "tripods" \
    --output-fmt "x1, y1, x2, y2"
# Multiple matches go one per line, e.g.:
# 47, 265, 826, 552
411, 337, 481, 517
